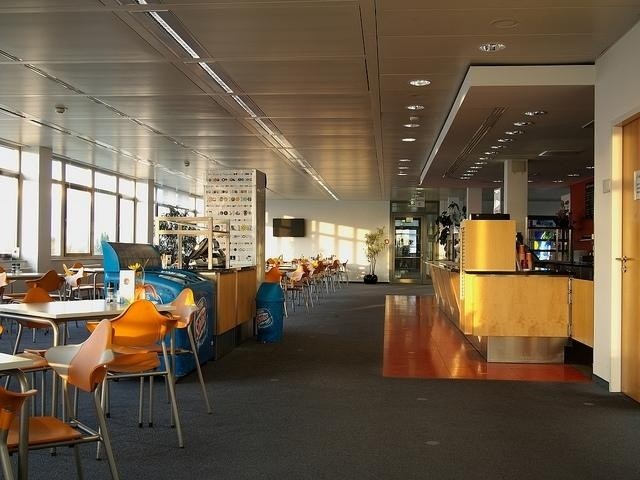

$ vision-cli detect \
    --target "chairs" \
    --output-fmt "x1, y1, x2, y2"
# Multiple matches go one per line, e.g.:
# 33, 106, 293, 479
0, 261, 106, 343
1, 387, 37, 480
264, 253, 349, 319
7, 318, 119, 479
139, 287, 212, 427
87, 299, 184, 460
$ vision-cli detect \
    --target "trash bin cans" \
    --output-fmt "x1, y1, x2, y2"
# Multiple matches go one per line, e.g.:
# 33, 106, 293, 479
256, 283, 285, 343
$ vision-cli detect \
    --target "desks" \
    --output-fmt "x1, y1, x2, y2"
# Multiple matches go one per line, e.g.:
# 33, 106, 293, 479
2, 298, 177, 457
1, 352, 34, 478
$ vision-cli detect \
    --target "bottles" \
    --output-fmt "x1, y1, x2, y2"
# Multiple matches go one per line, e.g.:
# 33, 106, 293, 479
106, 282, 115, 303
11, 263, 20, 274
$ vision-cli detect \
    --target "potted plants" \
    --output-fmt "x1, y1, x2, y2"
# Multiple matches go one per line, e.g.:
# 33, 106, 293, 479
363, 226, 386, 286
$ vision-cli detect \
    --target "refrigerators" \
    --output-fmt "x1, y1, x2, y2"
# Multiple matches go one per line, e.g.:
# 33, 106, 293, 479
103, 243, 218, 382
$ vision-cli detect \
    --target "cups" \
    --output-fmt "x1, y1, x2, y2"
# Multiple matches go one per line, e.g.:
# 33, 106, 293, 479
519, 245, 533, 269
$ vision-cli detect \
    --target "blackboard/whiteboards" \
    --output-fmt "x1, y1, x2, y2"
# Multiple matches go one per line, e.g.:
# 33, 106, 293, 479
585, 181, 594, 219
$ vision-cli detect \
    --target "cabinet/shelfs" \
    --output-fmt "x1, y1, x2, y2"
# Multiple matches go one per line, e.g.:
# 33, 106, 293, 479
529, 216, 566, 261
197, 266, 258, 359
